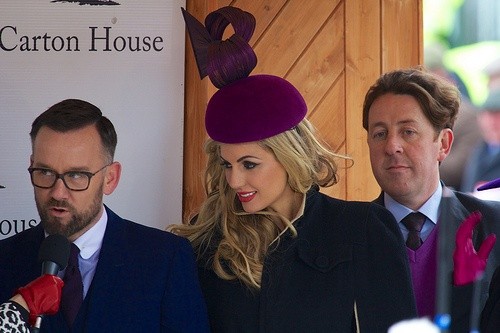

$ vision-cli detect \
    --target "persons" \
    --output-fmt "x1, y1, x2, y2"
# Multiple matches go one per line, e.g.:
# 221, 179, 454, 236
0, 272, 65, 333
168, 75, 418, 333
0, 100, 212, 333
363, 68, 500, 333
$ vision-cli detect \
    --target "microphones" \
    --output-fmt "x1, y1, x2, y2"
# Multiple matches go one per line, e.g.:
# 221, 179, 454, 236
32, 233, 71, 333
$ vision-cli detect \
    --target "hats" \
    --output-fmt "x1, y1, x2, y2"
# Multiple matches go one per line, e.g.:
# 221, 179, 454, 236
181, 7, 308, 144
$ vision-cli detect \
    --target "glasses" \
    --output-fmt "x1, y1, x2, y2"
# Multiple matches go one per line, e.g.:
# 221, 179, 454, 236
28, 163, 111, 191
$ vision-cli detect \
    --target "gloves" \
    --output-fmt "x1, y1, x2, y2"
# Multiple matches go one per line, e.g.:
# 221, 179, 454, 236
453, 210, 497, 284
12, 273, 64, 326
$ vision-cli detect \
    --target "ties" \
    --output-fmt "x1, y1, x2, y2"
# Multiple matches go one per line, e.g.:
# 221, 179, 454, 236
400, 212, 427, 251
62, 243, 84, 329
